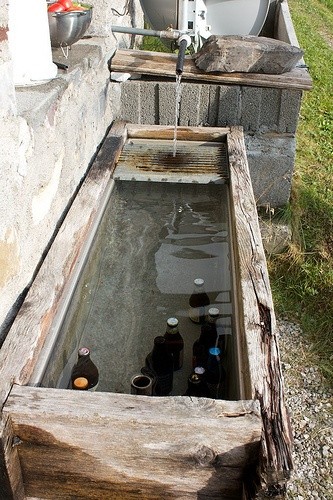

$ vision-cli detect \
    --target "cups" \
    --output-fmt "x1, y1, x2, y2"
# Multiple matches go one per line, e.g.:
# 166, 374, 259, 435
130, 375, 153, 397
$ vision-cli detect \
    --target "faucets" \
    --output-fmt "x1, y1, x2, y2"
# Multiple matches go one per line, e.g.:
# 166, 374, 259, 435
175, 34, 191, 75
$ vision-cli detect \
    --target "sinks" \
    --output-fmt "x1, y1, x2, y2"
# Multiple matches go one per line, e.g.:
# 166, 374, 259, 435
0, 120, 295, 500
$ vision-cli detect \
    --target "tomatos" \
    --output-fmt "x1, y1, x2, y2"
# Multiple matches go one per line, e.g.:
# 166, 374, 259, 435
48, 0, 85, 12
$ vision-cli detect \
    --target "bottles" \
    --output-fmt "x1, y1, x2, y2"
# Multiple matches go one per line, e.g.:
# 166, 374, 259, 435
73, 376, 90, 391
145, 335, 174, 396
188, 278, 210, 324
207, 307, 225, 358
71, 347, 99, 392
191, 326, 217, 367
181, 365, 215, 398
206, 346, 226, 399
162, 318, 184, 370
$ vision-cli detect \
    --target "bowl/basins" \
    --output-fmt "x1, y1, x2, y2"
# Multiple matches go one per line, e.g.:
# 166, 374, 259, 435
47, 4, 95, 48
